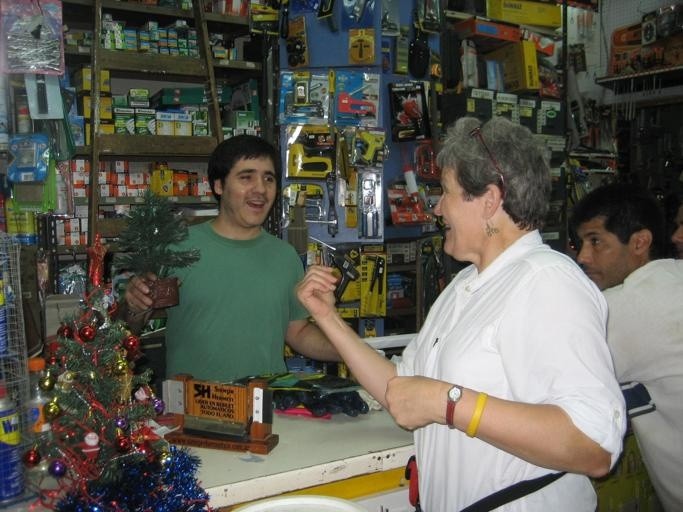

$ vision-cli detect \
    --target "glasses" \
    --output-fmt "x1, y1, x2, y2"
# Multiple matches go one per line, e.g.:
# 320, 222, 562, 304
470, 118, 497, 166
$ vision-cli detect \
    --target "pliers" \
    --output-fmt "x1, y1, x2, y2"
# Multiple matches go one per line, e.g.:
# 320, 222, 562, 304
365, 255, 385, 317
361, 179, 379, 237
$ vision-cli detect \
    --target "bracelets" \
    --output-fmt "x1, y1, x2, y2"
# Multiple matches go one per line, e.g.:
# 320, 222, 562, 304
126, 307, 144, 318
465, 393, 488, 437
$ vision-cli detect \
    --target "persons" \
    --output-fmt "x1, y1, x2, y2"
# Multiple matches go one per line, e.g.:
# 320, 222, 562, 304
573, 184, 683, 511
113, 134, 344, 383
292, 114, 629, 511
671, 203, 683, 261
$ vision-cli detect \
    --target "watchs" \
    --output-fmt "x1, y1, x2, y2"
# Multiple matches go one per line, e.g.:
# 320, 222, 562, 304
446, 384, 463, 429
